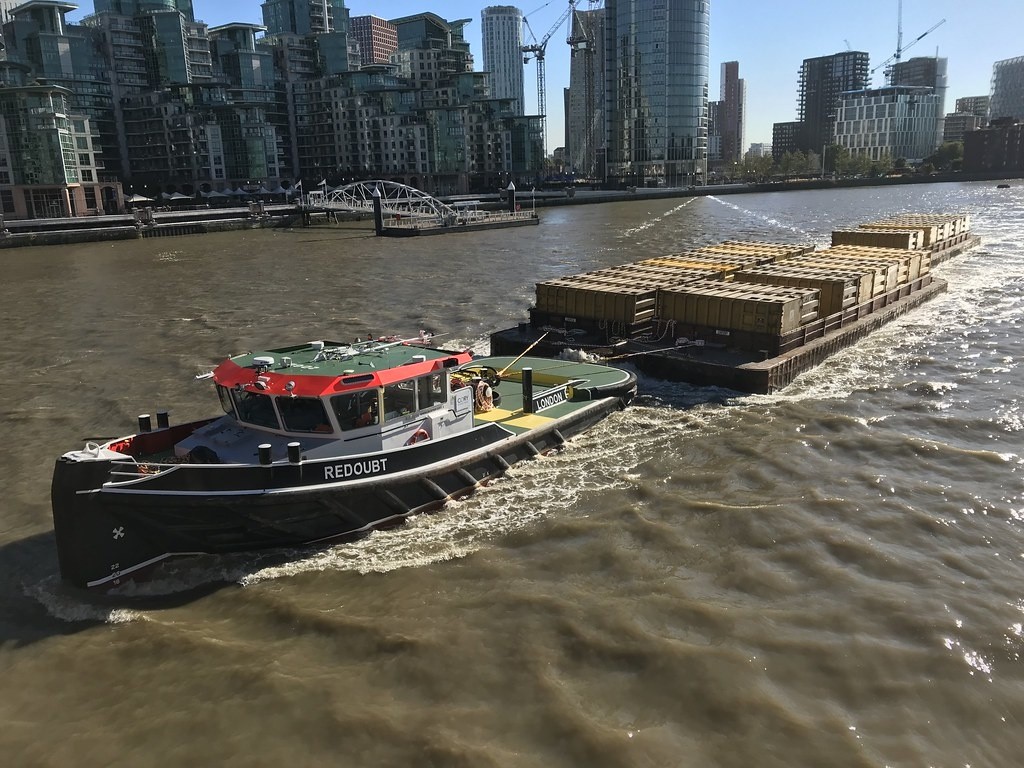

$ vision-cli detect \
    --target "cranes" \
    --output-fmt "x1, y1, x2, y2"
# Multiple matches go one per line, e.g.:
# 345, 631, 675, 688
870, 0, 949, 76
520, 0, 607, 190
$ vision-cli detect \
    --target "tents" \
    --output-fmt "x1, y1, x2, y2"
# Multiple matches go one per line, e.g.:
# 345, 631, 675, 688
124, 185, 303, 208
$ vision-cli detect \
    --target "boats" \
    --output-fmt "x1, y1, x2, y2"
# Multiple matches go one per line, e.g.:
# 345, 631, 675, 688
49, 330, 639, 591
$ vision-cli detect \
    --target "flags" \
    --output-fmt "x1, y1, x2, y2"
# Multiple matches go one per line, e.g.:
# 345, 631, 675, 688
295, 180, 301, 189
318, 180, 325, 185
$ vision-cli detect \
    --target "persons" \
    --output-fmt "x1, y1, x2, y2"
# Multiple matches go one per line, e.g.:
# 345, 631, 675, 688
368, 333, 373, 341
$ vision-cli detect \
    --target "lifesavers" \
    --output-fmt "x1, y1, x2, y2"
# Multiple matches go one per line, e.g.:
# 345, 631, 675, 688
413, 427, 431, 446
